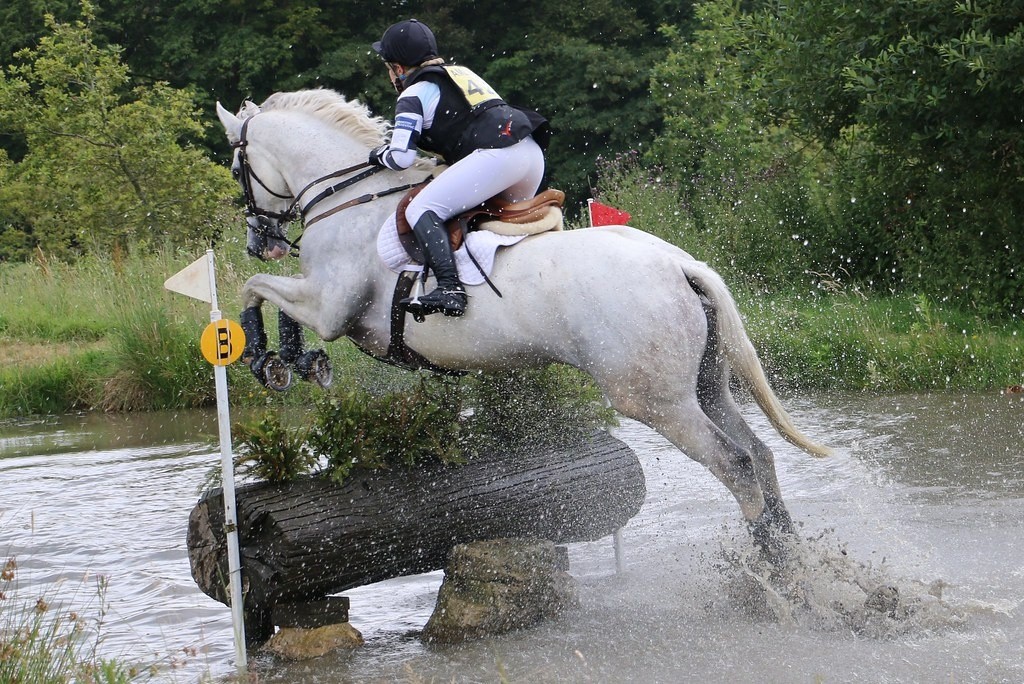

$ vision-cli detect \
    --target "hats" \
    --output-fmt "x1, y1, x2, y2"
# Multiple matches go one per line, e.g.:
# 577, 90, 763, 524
371, 18, 439, 66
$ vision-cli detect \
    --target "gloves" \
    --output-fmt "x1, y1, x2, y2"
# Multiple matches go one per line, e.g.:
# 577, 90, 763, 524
367, 144, 390, 166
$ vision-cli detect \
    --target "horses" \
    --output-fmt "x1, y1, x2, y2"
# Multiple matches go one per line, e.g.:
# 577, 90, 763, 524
215, 86, 829, 571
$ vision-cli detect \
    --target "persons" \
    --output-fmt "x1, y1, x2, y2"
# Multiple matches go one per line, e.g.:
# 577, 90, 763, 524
368, 19, 551, 318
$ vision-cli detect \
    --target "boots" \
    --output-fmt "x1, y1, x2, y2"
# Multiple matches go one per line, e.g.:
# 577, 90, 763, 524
398, 210, 472, 316
